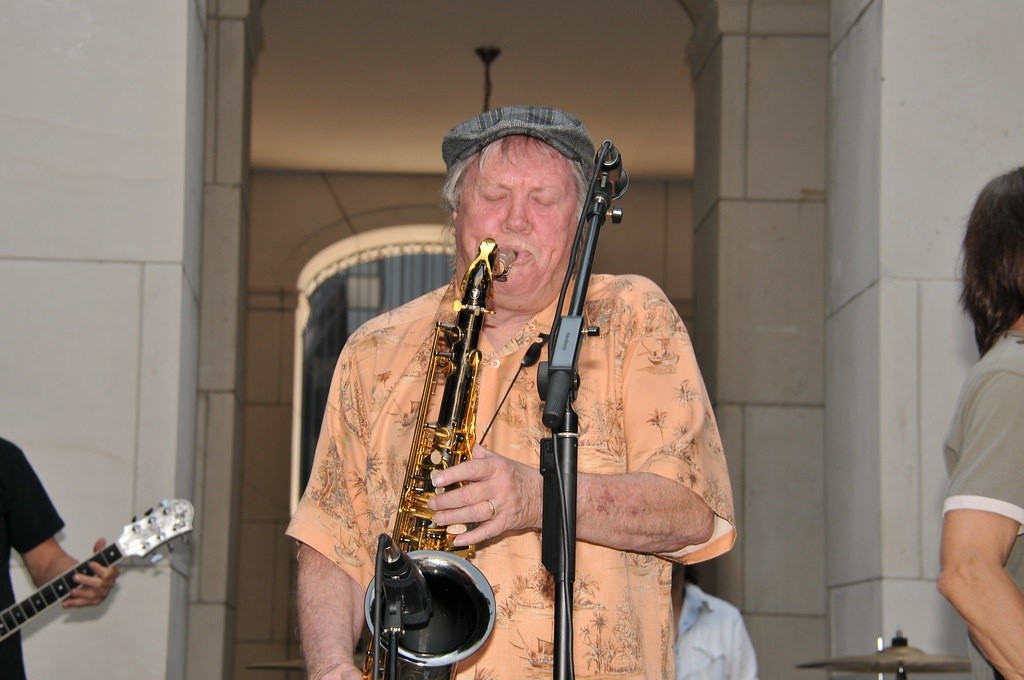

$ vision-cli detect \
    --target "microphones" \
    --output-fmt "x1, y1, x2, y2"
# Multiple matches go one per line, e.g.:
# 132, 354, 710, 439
593, 139, 629, 202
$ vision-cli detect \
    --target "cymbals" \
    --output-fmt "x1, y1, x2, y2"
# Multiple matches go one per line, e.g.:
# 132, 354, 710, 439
794, 646, 971, 673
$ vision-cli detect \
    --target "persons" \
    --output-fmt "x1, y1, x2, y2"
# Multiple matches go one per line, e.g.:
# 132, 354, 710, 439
934, 165, 1024, 679
663, 560, 760, 680
0, 437, 119, 680
285, 104, 741, 679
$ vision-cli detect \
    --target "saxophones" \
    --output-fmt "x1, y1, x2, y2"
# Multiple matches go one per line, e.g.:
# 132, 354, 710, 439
358, 239, 516, 680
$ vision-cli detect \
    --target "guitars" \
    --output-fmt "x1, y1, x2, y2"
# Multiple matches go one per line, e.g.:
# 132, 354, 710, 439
0, 496, 196, 648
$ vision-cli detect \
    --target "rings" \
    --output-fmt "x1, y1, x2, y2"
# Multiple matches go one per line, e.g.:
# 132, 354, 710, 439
486, 499, 496, 518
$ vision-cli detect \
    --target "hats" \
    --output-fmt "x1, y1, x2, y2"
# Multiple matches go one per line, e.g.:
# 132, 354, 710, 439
441, 104, 596, 178
